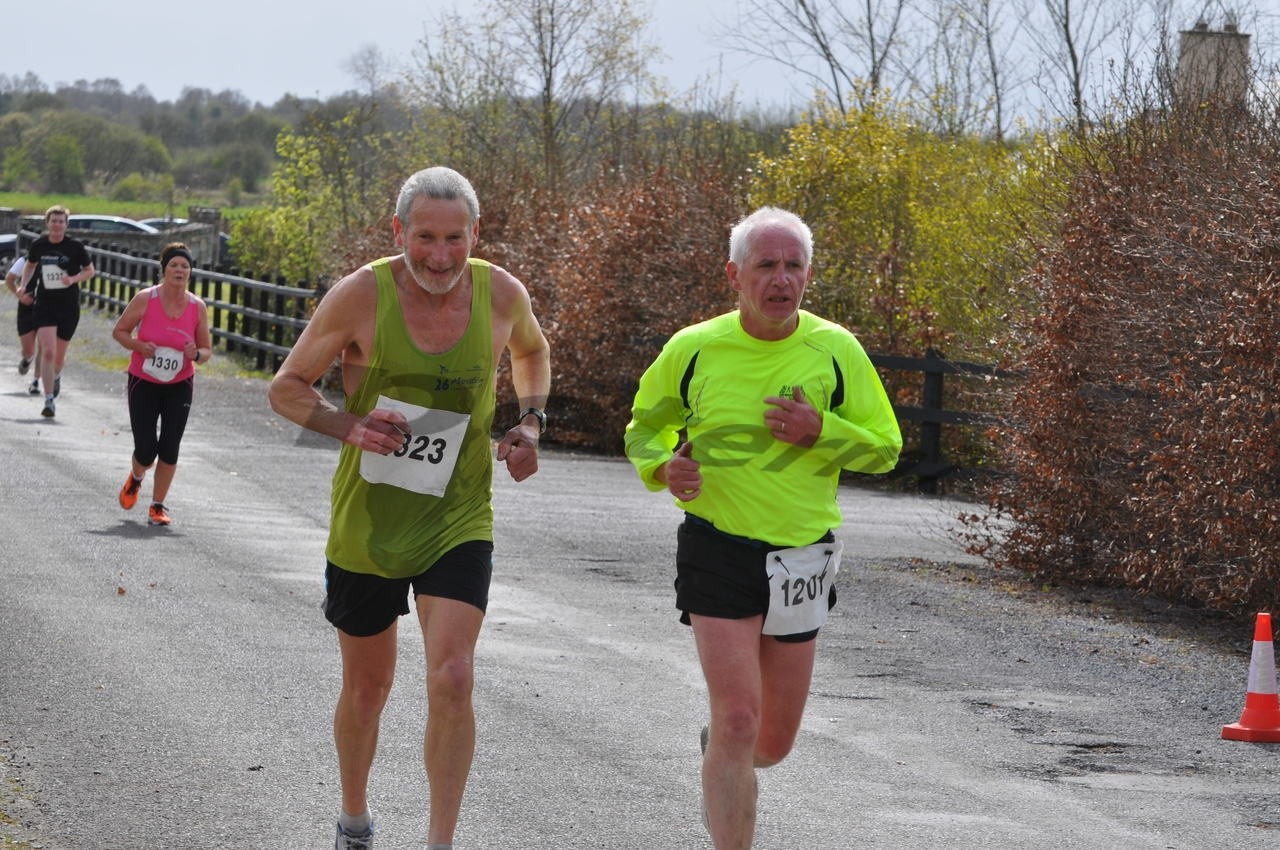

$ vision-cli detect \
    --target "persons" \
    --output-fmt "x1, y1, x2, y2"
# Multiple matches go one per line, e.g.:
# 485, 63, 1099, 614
17, 204, 95, 415
625, 207, 904, 850
111, 241, 211, 524
267, 165, 552, 850
6, 239, 42, 393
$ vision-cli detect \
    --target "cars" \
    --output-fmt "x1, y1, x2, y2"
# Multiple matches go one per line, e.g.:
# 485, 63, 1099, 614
0, 214, 233, 281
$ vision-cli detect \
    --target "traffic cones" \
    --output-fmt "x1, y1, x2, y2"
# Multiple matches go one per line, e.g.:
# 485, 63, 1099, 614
1221, 612, 1280, 743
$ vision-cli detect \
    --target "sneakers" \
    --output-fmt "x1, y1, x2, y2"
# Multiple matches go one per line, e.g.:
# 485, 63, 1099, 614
335, 819, 374, 850
147, 504, 170, 525
120, 470, 145, 510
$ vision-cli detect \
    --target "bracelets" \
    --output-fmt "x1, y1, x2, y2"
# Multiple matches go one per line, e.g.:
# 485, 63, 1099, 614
194, 350, 201, 362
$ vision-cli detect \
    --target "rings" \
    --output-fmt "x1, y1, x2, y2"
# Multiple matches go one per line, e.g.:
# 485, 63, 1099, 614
780, 421, 785, 433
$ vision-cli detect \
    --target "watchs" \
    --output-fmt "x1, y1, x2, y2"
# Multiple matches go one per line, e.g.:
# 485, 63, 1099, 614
519, 408, 548, 434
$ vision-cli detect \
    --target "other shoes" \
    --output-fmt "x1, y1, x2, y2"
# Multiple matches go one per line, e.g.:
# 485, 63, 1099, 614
18, 354, 34, 374
41, 399, 55, 417
29, 380, 40, 393
54, 377, 60, 397
